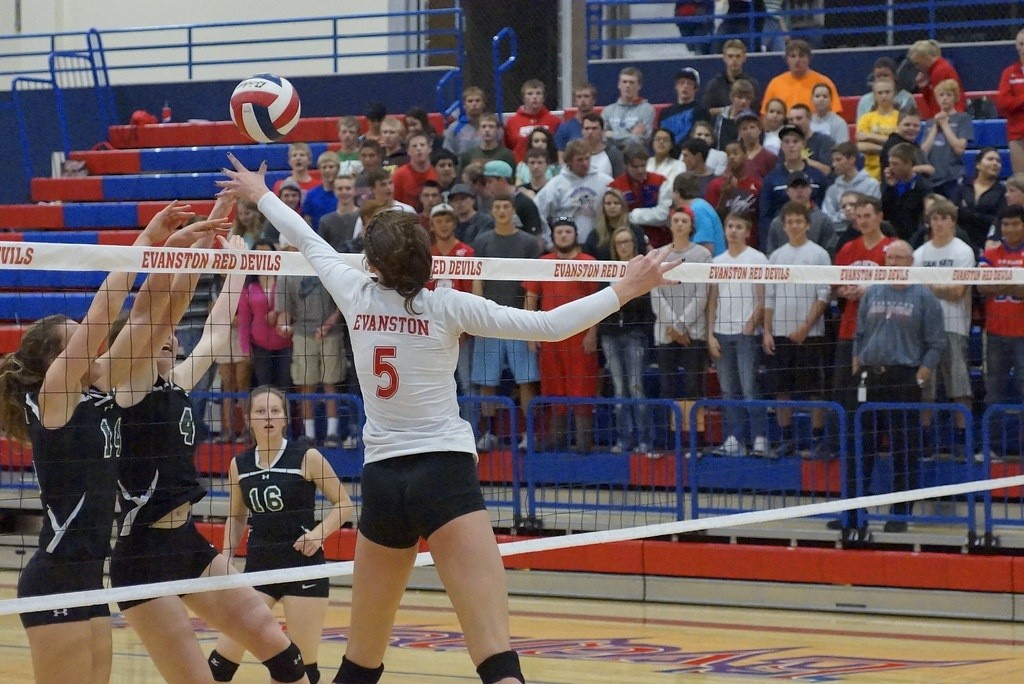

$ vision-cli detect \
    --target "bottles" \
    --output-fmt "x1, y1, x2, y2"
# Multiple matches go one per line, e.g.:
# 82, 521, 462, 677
49, 148, 63, 178
964, 96, 999, 120
857, 371, 868, 403
161, 97, 171, 123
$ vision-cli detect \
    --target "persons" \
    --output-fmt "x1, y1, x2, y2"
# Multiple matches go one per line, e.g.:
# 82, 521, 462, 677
762, 0, 791, 53
596, 229, 659, 456
423, 204, 478, 411
470, 193, 532, 451
975, 201, 1024, 456
709, 0, 765, 54
109, 182, 310, 684
518, 215, 596, 452
763, 200, 835, 459
836, 196, 893, 404
202, 384, 353, 684
0, 200, 233, 684
205, 24, 1024, 408
705, 212, 774, 456
212, 145, 688, 684
672, 0, 714, 55
647, 205, 714, 459
826, 240, 948, 532
909, 198, 973, 462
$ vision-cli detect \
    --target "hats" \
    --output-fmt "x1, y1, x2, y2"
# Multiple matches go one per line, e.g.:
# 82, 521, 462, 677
551, 216, 576, 229
734, 110, 760, 127
786, 171, 811, 188
429, 203, 455, 221
779, 124, 804, 141
669, 204, 694, 223
676, 67, 701, 88
447, 184, 478, 201
279, 176, 301, 192
481, 160, 513, 179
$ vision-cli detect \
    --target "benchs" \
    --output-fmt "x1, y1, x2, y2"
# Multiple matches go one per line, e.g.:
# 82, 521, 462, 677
0, 94, 1023, 499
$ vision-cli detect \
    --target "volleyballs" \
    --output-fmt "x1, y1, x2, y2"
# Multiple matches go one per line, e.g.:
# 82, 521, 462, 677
228, 71, 302, 146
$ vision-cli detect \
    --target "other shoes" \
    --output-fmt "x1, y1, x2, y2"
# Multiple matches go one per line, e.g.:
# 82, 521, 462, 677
826, 518, 841, 530
884, 520, 909, 533
192, 414, 1023, 464
